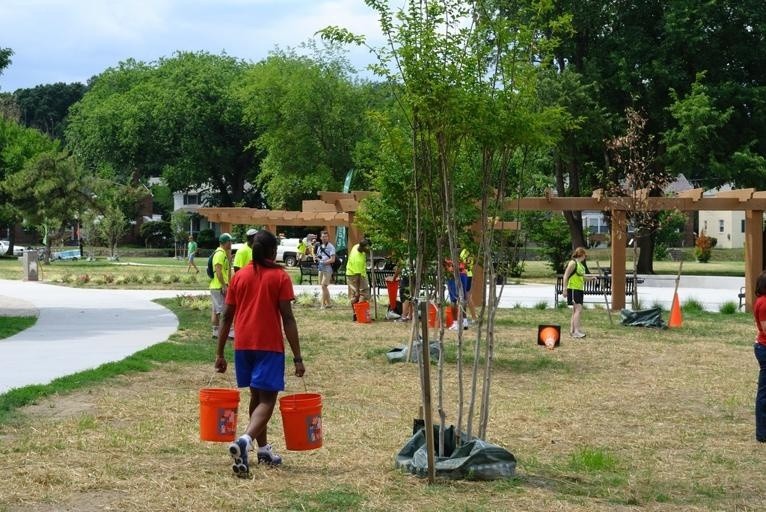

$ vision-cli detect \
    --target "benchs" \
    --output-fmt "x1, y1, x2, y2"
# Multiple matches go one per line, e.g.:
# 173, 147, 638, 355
298, 260, 348, 288
555, 274, 645, 311
362, 266, 445, 301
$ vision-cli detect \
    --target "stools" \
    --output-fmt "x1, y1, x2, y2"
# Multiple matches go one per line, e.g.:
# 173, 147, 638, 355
737, 285, 746, 312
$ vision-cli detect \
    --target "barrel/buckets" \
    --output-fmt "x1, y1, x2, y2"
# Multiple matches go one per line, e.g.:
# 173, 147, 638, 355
279, 394, 323, 450
200, 389, 240, 442
428, 303, 453, 327
448, 273, 468, 302
354, 301, 371, 323
386, 276, 401, 311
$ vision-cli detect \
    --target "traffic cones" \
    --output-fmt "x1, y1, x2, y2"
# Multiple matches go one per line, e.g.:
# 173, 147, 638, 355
383, 276, 400, 311
536, 324, 562, 351
667, 293, 684, 328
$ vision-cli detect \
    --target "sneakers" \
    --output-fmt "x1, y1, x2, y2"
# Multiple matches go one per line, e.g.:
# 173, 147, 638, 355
448, 322, 469, 330
257, 448, 283, 465
228, 438, 249, 478
386, 310, 401, 319
568, 332, 587, 338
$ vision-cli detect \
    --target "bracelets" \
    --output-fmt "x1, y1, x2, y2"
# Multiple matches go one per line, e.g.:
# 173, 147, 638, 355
293, 358, 302, 363
563, 288, 567, 291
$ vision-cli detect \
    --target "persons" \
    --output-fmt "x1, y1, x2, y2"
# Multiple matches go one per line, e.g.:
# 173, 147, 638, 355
460, 247, 478, 324
345, 240, 372, 321
387, 256, 416, 323
187, 236, 200, 274
233, 227, 335, 339
753, 271, 766, 443
562, 246, 597, 339
214, 231, 306, 479
444, 257, 468, 330
207, 233, 236, 339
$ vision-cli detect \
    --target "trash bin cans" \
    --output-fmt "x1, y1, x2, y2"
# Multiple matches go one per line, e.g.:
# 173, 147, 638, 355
492, 263, 508, 285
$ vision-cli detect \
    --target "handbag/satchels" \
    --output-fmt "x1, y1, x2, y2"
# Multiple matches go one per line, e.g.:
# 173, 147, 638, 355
331, 256, 342, 272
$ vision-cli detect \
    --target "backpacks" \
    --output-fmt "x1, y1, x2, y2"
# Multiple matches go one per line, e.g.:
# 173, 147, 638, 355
206, 250, 225, 279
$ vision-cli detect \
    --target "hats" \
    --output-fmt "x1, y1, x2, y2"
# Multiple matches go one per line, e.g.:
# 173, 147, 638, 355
219, 232, 236, 243
247, 229, 258, 235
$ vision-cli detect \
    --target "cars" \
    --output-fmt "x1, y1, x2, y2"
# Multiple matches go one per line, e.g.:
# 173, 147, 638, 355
0, 240, 25, 258
230, 232, 312, 268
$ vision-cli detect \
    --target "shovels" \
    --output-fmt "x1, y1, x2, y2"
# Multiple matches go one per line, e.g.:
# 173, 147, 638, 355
413, 406, 425, 437
